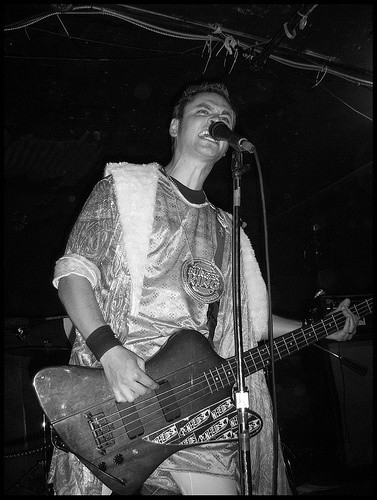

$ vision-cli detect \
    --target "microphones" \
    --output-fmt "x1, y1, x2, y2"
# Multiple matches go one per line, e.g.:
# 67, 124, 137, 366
209, 121, 256, 153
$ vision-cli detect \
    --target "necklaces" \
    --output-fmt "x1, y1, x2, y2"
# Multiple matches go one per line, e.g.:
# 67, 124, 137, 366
161, 162, 226, 304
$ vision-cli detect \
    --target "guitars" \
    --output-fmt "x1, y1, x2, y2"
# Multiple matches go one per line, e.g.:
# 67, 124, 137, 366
31, 295, 374, 495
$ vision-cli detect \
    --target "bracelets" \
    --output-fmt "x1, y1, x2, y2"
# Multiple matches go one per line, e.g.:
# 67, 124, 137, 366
86, 324, 122, 362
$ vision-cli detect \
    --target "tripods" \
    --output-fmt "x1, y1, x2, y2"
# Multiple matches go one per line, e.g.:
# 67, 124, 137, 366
2, 411, 55, 496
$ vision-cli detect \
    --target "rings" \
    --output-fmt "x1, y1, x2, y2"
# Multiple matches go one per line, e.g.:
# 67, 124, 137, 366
349, 333, 352, 334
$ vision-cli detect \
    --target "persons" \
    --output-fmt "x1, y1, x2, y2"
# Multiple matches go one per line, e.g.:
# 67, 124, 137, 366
45, 79, 360, 495
